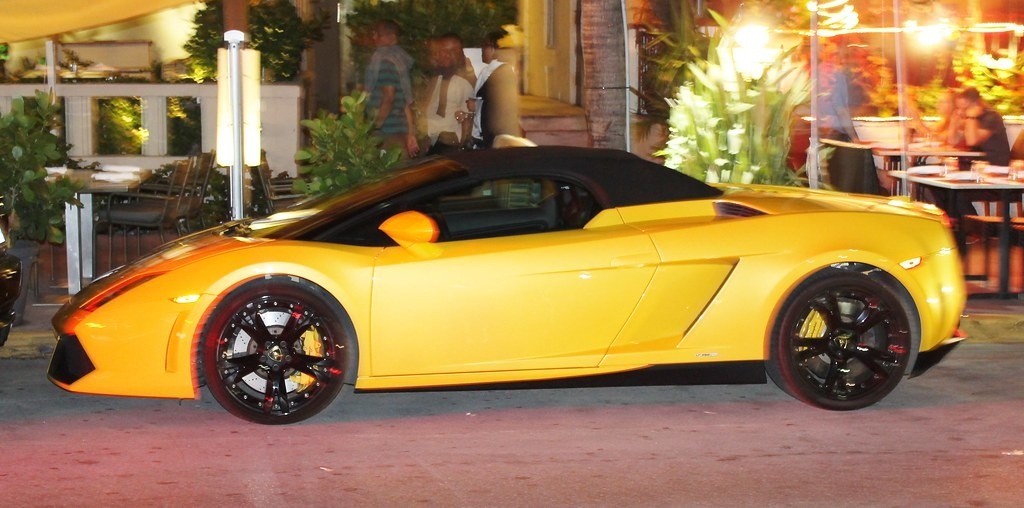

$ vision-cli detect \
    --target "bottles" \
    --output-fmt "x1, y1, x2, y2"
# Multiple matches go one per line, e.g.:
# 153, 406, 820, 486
71, 60, 78, 82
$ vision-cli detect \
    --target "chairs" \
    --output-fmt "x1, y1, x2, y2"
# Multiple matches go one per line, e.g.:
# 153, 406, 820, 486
94, 148, 218, 272
556, 186, 596, 229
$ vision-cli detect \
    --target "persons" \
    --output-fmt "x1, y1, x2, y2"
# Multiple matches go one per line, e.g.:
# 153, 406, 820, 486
905, 86, 1010, 169
814, 35, 868, 163
341, 19, 518, 162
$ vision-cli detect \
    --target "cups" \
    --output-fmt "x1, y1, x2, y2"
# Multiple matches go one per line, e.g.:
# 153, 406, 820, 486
1008, 160, 1024, 183
940, 157, 959, 179
971, 161, 989, 184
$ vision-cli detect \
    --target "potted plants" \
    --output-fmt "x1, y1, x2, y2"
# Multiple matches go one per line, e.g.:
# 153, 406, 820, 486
0, 87, 103, 323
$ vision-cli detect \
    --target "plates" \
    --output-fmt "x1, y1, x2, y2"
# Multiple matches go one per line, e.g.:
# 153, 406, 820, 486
908, 165, 957, 174
945, 171, 977, 181
91, 172, 138, 183
95, 166, 139, 172
985, 165, 1024, 178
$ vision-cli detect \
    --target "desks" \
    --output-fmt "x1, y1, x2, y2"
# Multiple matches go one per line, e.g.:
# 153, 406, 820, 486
31, 165, 153, 308
868, 144, 989, 165
889, 167, 1024, 301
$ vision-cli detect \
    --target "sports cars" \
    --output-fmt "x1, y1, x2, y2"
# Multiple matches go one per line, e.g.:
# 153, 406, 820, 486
44, 144, 968, 426
